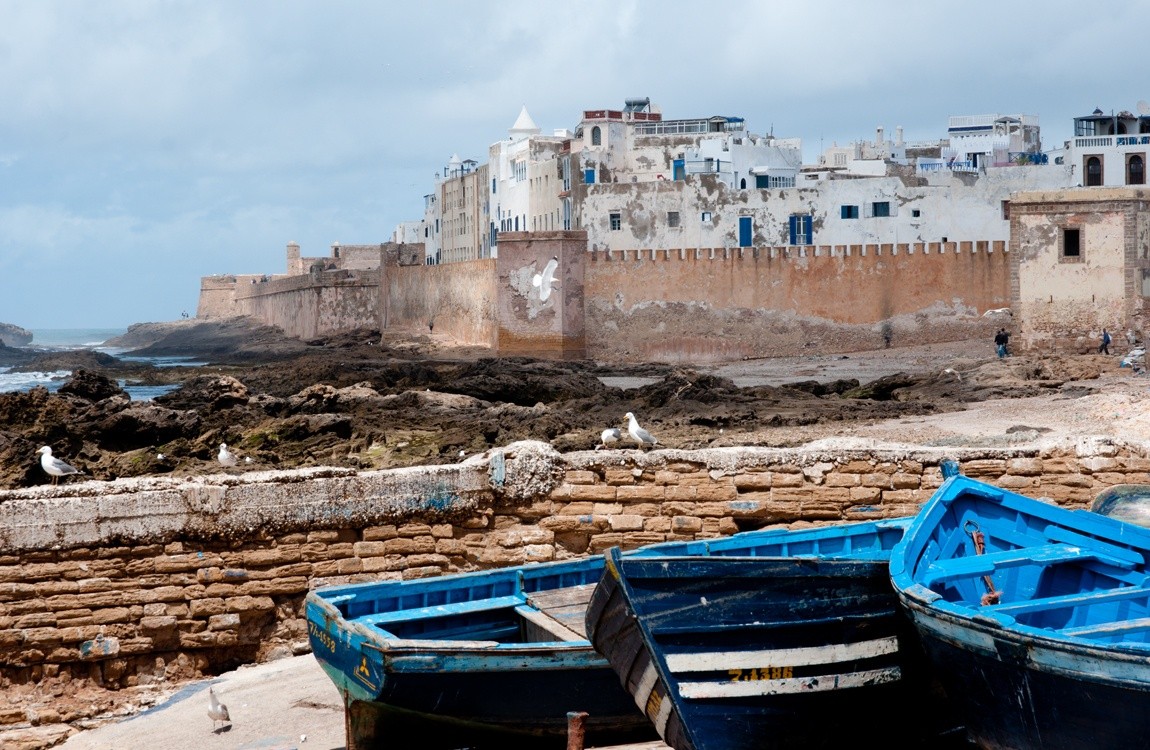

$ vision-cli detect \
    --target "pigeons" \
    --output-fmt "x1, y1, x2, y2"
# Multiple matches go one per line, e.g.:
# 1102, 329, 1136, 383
219, 442, 242, 476
624, 412, 658, 450
36, 445, 79, 485
206, 686, 230, 732
601, 429, 621, 450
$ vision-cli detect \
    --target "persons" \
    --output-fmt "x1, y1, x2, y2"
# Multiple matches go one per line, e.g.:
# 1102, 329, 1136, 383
1098, 328, 1110, 355
993, 327, 1010, 359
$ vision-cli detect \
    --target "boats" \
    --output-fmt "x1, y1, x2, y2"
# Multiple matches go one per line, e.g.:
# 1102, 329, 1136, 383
305, 516, 914, 749
1089, 481, 1150, 531
887, 459, 1150, 750
583, 544, 984, 750
